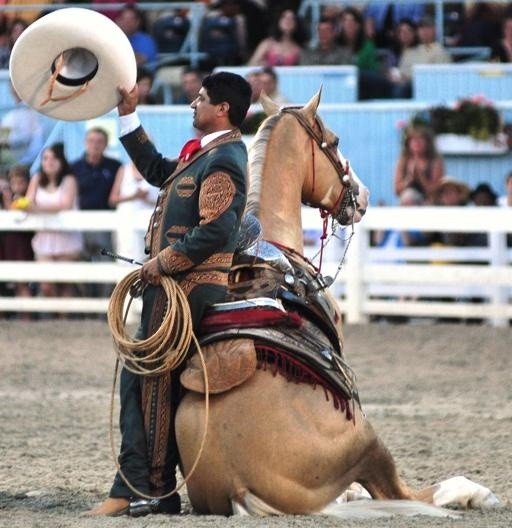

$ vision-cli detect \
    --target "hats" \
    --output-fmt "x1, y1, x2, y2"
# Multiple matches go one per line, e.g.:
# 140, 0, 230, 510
8, 8, 138, 121
470, 183, 497, 201
429, 176, 470, 204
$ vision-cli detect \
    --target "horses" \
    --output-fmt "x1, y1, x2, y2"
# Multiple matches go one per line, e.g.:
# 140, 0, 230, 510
169, 79, 505, 524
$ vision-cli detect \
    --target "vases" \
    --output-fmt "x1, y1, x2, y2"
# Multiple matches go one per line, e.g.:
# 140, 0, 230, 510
430, 133, 508, 156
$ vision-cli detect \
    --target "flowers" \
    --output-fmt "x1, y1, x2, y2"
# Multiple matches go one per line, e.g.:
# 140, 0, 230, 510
396, 92, 505, 145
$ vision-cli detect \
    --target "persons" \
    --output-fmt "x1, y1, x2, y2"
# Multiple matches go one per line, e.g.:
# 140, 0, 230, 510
180, 71, 209, 105
23, 142, 83, 302
245, 2, 512, 103
128, 71, 160, 106
86, 70, 251, 518
67, 125, 149, 258
374, 115, 511, 325
0, 166, 33, 322
113, 0, 245, 71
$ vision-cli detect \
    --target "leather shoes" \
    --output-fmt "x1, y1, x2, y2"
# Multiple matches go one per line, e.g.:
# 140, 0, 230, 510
79, 496, 130, 519
